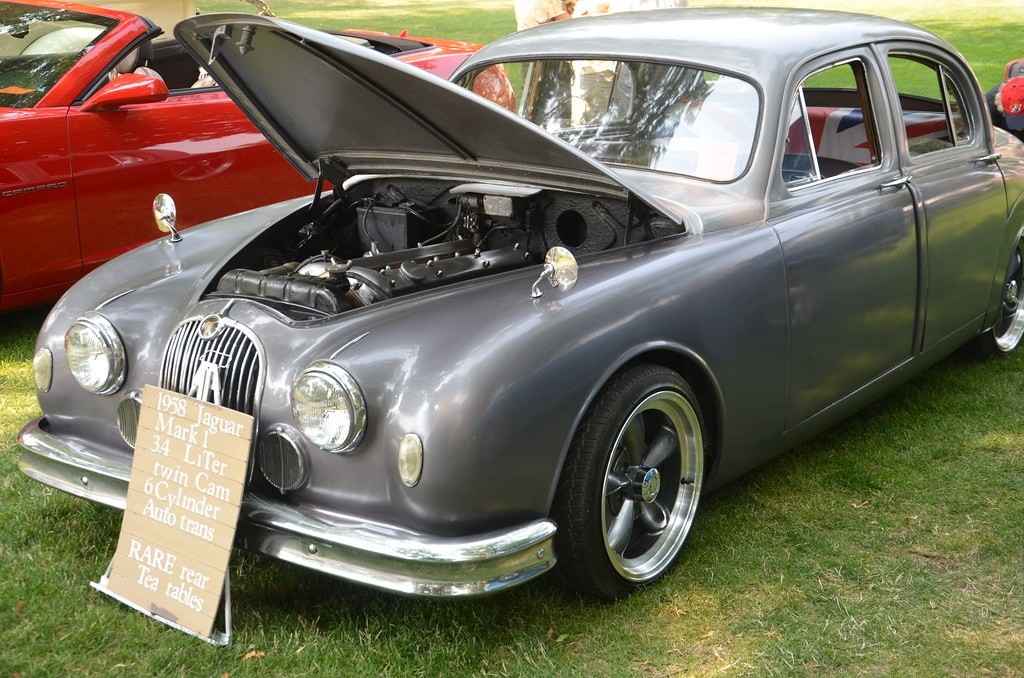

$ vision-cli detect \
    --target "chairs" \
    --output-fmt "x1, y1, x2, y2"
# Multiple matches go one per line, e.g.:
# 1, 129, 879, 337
731, 150, 858, 184
113, 39, 153, 78
570, 141, 669, 166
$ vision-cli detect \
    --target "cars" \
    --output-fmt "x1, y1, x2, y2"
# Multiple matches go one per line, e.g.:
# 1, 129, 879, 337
16, 11, 1024, 604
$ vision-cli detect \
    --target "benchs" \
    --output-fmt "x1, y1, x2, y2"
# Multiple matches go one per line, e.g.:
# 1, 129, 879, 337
664, 97, 967, 166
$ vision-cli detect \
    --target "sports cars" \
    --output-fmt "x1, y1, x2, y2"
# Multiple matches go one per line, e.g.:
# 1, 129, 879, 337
0, 0, 518, 311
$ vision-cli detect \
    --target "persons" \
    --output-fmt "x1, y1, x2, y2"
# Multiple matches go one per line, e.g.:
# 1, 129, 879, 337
984, 75, 1024, 144
512, 0, 688, 126
